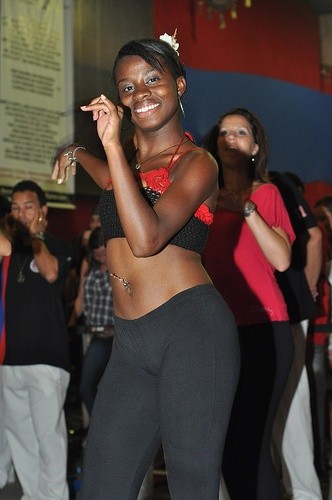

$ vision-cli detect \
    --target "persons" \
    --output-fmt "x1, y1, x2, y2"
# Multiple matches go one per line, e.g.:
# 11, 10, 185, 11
0, 110, 332, 500
51, 39, 239, 500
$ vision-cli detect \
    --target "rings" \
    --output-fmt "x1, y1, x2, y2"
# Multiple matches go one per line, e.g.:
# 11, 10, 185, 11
38, 217, 43, 222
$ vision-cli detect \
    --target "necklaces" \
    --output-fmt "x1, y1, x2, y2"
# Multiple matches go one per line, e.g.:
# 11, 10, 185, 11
134, 139, 189, 170
15, 249, 30, 282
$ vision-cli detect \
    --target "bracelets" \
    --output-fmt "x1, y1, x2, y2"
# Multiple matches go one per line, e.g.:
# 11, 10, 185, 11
63, 146, 86, 161
244, 200, 256, 217
29, 234, 46, 241
